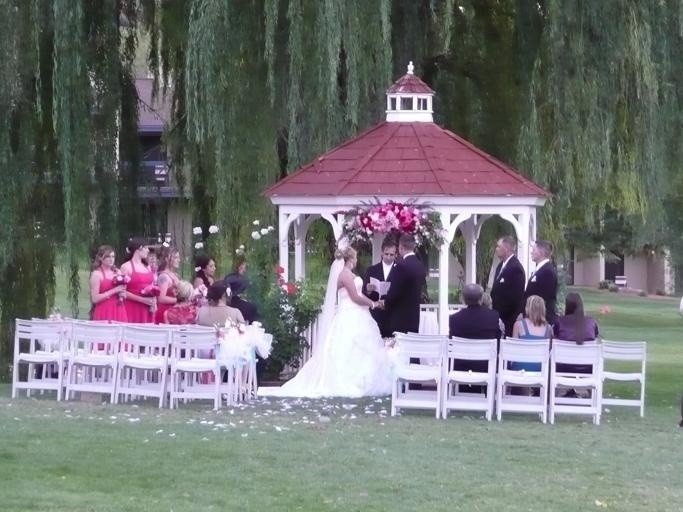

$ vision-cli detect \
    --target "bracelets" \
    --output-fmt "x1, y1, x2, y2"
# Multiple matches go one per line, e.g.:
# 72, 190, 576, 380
371, 301, 375, 310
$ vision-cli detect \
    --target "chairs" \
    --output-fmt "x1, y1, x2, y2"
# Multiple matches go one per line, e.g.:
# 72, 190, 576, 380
385, 328, 648, 426
8, 312, 262, 412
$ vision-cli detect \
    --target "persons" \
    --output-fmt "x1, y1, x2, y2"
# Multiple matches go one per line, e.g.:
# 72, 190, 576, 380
554, 292, 600, 397
378, 235, 426, 390
516, 240, 557, 323
490, 236, 526, 337
677, 296, 683, 427
254, 237, 399, 397
509, 295, 554, 397
449, 283, 501, 394
363, 237, 398, 333
89, 235, 265, 383
482, 293, 505, 337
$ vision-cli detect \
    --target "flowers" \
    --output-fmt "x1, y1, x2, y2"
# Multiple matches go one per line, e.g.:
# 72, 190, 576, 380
111, 273, 132, 289
191, 283, 208, 306
177, 283, 192, 301
326, 193, 446, 253
141, 284, 161, 300
